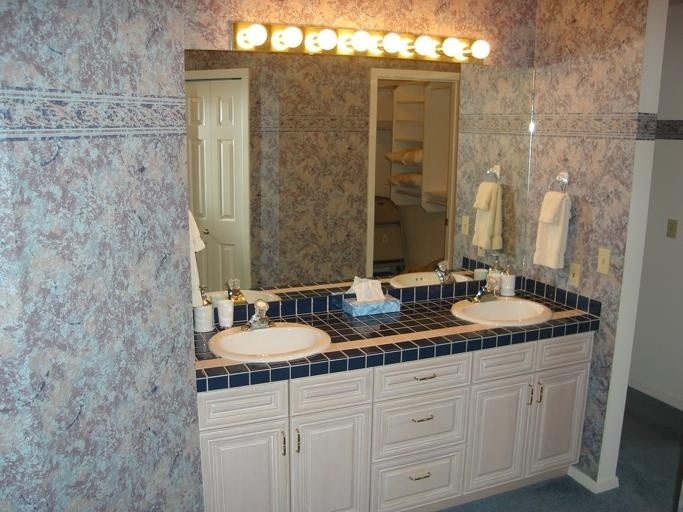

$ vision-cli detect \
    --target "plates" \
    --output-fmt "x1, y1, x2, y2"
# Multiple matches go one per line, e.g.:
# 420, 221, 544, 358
196, 331, 595, 512
375, 79, 454, 214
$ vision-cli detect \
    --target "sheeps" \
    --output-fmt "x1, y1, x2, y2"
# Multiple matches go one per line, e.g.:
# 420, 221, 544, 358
468, 277, 496, 305
434, 260, 455, 284
240, 299, 273, 330
225, 277, 247, 304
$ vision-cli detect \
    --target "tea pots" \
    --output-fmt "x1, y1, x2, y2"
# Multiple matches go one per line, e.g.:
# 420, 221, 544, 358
184, 48, 535, 308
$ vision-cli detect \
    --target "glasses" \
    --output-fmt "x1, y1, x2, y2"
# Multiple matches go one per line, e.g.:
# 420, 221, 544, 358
233, 21, 490, 65
